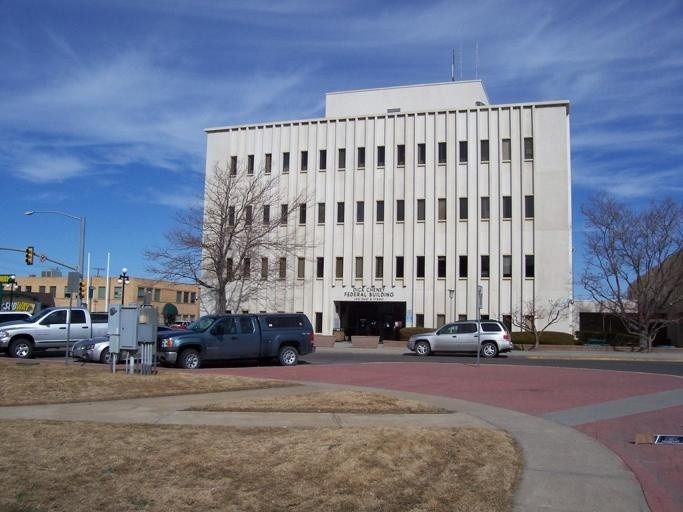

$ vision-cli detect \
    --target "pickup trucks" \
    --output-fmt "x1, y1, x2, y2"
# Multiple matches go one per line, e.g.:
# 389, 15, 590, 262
0, 307, 109, 358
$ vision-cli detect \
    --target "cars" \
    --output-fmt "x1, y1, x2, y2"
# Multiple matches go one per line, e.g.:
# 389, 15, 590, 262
71, 314, 316, 369
407, 320, 514, 358
1, 310, 33, 323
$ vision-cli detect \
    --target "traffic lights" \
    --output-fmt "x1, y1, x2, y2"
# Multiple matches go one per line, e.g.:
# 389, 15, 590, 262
25, 246, 34, 265
79, 282, 86, 299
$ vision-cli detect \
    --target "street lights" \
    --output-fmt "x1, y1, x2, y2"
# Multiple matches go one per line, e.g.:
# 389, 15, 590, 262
118, 268, 130, 305
448, 289, 455, 322
6, 275, 18, 310
24, 210, 86, 307
